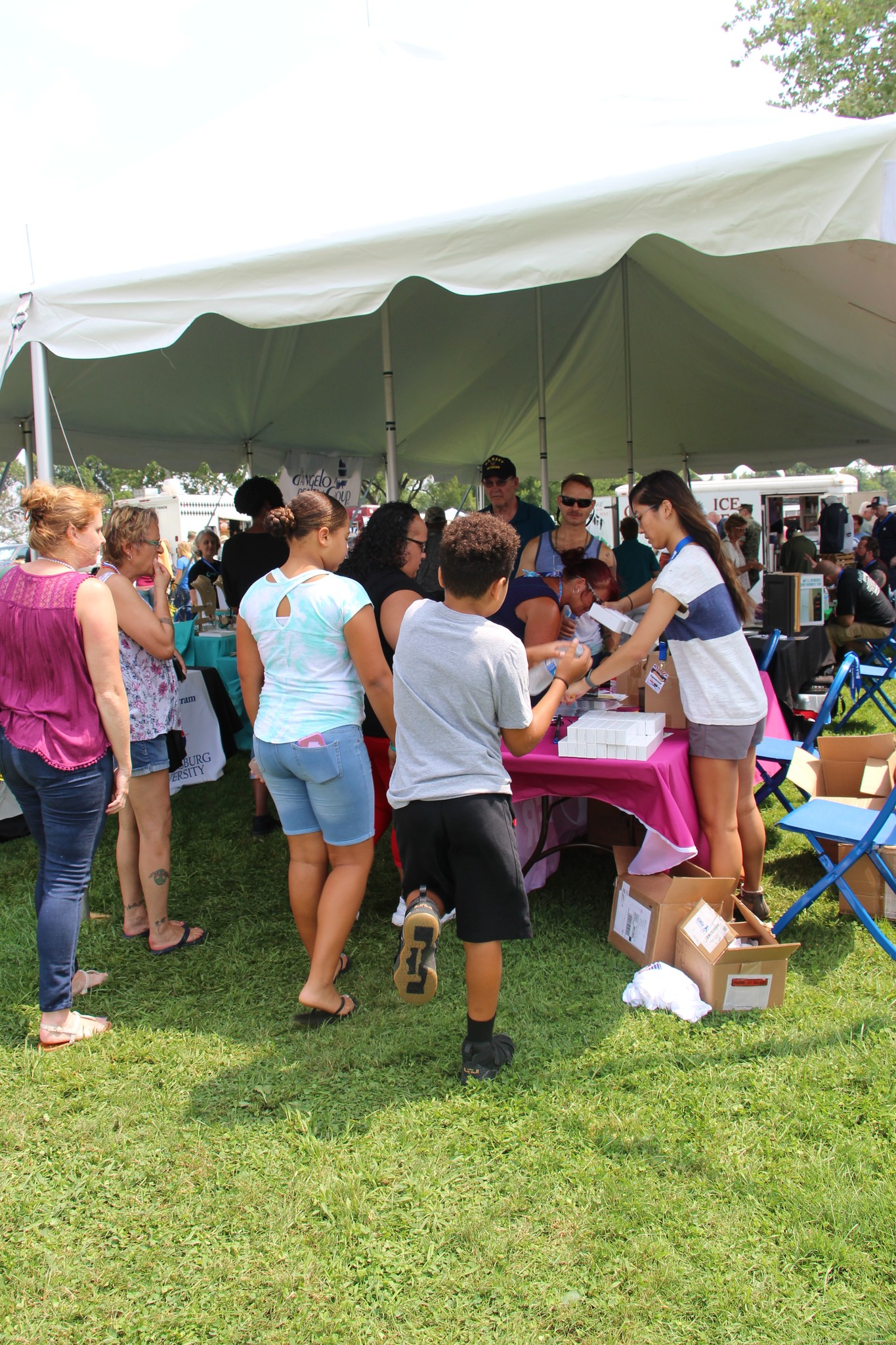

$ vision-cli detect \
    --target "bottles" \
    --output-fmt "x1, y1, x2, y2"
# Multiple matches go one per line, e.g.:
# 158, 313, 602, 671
824, 586, 829, 610
559, 608, 580, 638
527, 644, 584, 697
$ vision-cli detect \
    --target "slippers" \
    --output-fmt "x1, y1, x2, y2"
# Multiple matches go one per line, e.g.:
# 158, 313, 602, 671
294, 994, 363, 1029
120, 920, 206, 954
333, 953, 350, 984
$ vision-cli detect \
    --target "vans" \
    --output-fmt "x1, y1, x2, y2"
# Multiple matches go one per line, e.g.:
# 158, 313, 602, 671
587, 472, 858, 571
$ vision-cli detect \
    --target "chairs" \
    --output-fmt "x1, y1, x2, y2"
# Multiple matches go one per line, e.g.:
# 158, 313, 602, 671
758, 621, 896, 960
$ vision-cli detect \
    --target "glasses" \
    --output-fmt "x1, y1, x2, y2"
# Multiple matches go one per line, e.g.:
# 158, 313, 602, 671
406, 535, 428, 553
560, 495, 592, 508
853, 522, 858, 527
575, 575, 603, 606
138, 538, 162, 548
734, 526, 747, 532
634, 503, 656, 527
480, 478, 513, 489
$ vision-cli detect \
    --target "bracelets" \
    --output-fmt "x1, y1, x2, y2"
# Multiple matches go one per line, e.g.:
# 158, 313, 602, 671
626, 595, 633, 611
553, 676, 569, 690
390, 740, 396, 752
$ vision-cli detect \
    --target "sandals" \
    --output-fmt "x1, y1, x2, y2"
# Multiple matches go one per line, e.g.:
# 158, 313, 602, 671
71, 967, 109, 996
38, 1011, 114, 1053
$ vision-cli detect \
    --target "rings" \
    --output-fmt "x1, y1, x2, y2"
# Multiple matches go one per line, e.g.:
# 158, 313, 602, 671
155, 569, 161, 572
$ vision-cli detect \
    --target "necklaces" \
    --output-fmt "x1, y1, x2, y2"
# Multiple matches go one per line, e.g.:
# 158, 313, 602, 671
192, 580, 213, 597
216, 579, 223, 585
37, 556, 76, 573
101, 561, 121, 575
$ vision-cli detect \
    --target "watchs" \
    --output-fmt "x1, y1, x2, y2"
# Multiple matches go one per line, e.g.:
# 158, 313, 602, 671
585, 668, 600, 691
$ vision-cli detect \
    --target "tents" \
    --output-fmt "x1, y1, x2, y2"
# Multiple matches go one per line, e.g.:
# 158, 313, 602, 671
0, 113, 896, 503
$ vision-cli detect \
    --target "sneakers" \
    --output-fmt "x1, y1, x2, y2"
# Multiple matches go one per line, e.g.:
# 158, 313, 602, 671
394, 884, 440, 1003
461, 1034, 515, 1085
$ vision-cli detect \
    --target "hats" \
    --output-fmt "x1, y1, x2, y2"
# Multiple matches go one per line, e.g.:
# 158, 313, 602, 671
736, 503, 752, 511
866, 496, 888, 508
482, 455, 517, 480
786, 525, 803, 539
424, 507, 447, 524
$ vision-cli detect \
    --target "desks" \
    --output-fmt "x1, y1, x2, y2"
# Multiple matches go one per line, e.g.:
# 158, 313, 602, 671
0, 666, 245, 821
194, 628, 254, 750
500, 671, 792, 880
742, 619, 836, 740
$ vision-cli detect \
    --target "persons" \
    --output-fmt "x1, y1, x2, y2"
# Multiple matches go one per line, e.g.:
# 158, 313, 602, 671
0, 481, 133, 1053
93, 505, 207, 955
137, 454, 896, 1085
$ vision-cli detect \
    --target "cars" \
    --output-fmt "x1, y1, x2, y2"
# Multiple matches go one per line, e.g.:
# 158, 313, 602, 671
0, 543, 30, 573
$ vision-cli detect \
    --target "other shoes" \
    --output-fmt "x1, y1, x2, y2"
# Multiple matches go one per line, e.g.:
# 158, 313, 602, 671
392, 897, 458, 927
251, 815, 283, 836
733, 883, 771, 920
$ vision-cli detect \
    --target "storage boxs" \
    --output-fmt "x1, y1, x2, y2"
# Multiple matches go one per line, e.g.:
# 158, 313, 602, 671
785, 732, 896, 864
607, 845, 736, 968
558, 649, 690, 761
838, 843, 896, 922
674, 892, 801, 1014
